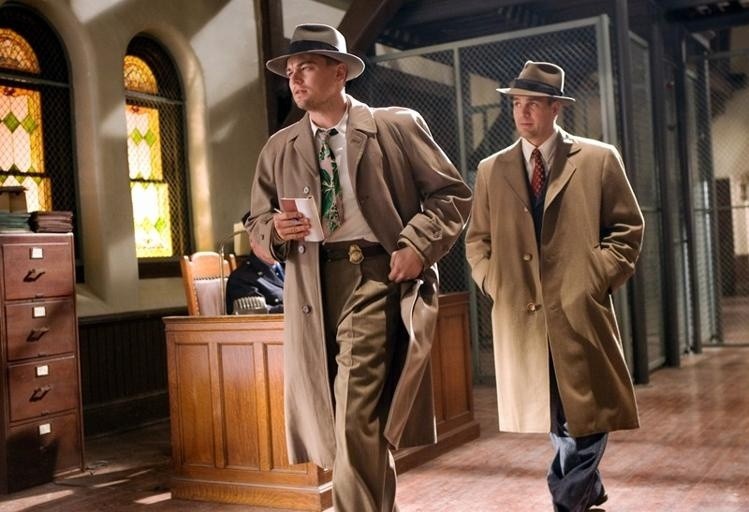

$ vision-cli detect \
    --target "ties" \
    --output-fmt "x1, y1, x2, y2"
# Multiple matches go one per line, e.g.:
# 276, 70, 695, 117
317, 129, 344, 237
530, 149, 545, 204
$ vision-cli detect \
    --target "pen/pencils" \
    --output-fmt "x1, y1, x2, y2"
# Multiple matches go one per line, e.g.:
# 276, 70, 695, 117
274, 208, 282, 213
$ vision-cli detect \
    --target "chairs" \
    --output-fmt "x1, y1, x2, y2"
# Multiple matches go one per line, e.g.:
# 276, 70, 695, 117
179, 251, 239, 315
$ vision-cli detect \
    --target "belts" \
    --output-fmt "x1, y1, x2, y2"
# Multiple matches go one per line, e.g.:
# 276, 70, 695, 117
319, 245, 385, 262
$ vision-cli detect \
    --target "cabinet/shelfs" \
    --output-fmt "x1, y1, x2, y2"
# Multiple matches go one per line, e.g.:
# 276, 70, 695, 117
1, 234, 89, 495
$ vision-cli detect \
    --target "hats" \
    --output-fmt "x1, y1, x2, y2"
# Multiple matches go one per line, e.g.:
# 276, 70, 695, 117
266, 24, 366, 82
496, 60, 576, 107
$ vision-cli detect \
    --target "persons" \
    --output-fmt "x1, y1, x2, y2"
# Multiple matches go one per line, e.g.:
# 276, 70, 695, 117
248, 23, 474, 512
228, 212, 286, 316
464, 57, 644, 512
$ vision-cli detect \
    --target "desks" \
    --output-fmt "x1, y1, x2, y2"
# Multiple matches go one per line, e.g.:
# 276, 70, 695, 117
164, 290, 483, 511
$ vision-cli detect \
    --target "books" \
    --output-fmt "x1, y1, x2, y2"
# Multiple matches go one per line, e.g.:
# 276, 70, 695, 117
0, 209, 74, 236
282, 196, 324, 244
